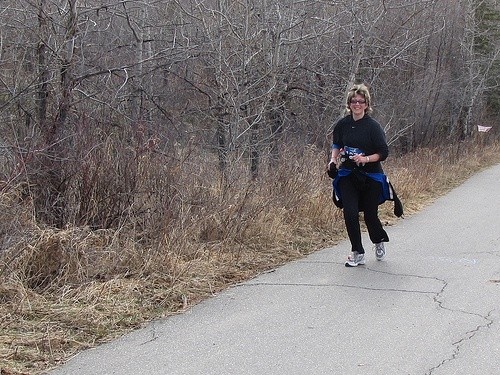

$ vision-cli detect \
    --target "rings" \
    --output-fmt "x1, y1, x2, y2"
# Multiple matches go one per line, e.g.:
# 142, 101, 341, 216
356, 159, 358, 162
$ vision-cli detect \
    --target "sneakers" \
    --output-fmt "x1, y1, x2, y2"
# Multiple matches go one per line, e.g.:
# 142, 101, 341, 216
344, 251, 365, 266
372, 242, 386, 260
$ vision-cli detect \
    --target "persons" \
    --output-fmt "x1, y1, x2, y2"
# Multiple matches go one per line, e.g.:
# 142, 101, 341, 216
326, 83, 390, 267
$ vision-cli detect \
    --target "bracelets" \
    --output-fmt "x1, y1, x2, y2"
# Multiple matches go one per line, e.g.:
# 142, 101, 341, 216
331, 156, 337, 158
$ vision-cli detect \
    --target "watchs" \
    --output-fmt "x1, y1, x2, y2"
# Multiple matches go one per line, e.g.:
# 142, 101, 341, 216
366, 156, 370, 163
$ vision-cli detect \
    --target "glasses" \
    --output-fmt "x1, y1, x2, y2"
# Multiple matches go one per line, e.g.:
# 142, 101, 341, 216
350, 98, 366, 104
